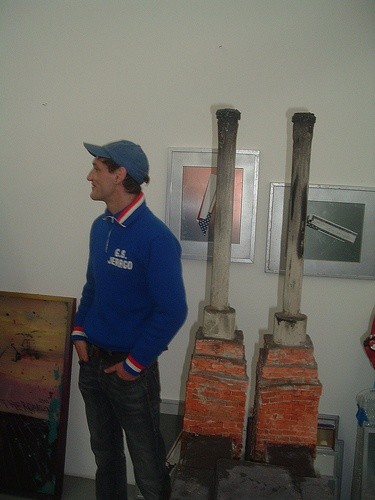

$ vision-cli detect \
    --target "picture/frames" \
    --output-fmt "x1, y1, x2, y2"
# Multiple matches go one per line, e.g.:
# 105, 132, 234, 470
264, 182, 375, 280
165, 147, 260, 263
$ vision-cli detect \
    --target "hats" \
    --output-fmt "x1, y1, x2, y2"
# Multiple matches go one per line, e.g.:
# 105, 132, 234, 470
83, 140, 150, 184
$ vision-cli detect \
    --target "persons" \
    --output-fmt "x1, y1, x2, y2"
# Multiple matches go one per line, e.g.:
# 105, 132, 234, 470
70, 140, 188, 500
